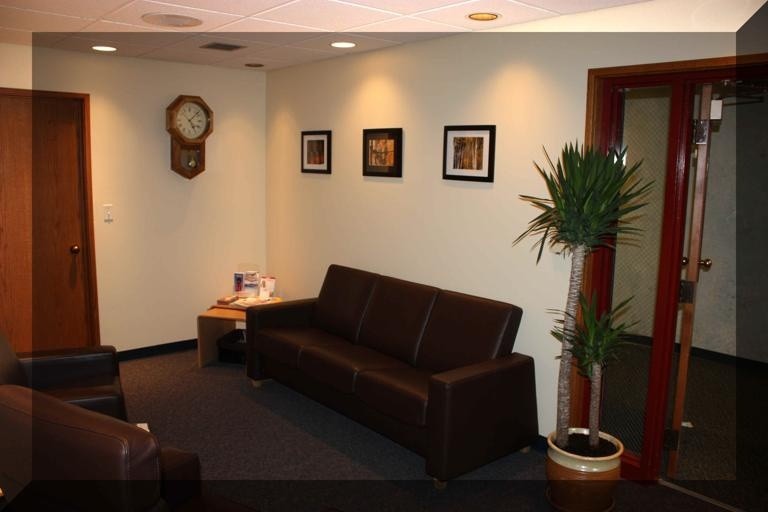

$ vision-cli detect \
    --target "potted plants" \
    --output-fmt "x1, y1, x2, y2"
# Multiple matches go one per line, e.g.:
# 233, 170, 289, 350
508, 136, 656, 505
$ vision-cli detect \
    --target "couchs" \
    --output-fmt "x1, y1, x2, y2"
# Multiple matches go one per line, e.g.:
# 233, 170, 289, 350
243, 262, 543, 488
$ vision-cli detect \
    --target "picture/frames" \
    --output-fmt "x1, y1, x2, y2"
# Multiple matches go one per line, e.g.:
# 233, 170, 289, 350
441, 124, 498, 182
300, 130, 333, 175
361, 128, 403, 177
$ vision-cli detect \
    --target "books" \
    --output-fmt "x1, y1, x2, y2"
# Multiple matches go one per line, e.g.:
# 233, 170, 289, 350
230, 296, 271, 312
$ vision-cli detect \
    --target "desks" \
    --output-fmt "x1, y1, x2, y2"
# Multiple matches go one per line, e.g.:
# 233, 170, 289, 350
196, 297, 282, 369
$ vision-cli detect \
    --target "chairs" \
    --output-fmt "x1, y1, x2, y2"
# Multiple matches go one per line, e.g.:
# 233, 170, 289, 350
0, 326, 204, 511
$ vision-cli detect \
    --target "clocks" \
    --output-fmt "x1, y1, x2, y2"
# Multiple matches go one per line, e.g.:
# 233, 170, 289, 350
167, 94, 215, 180
